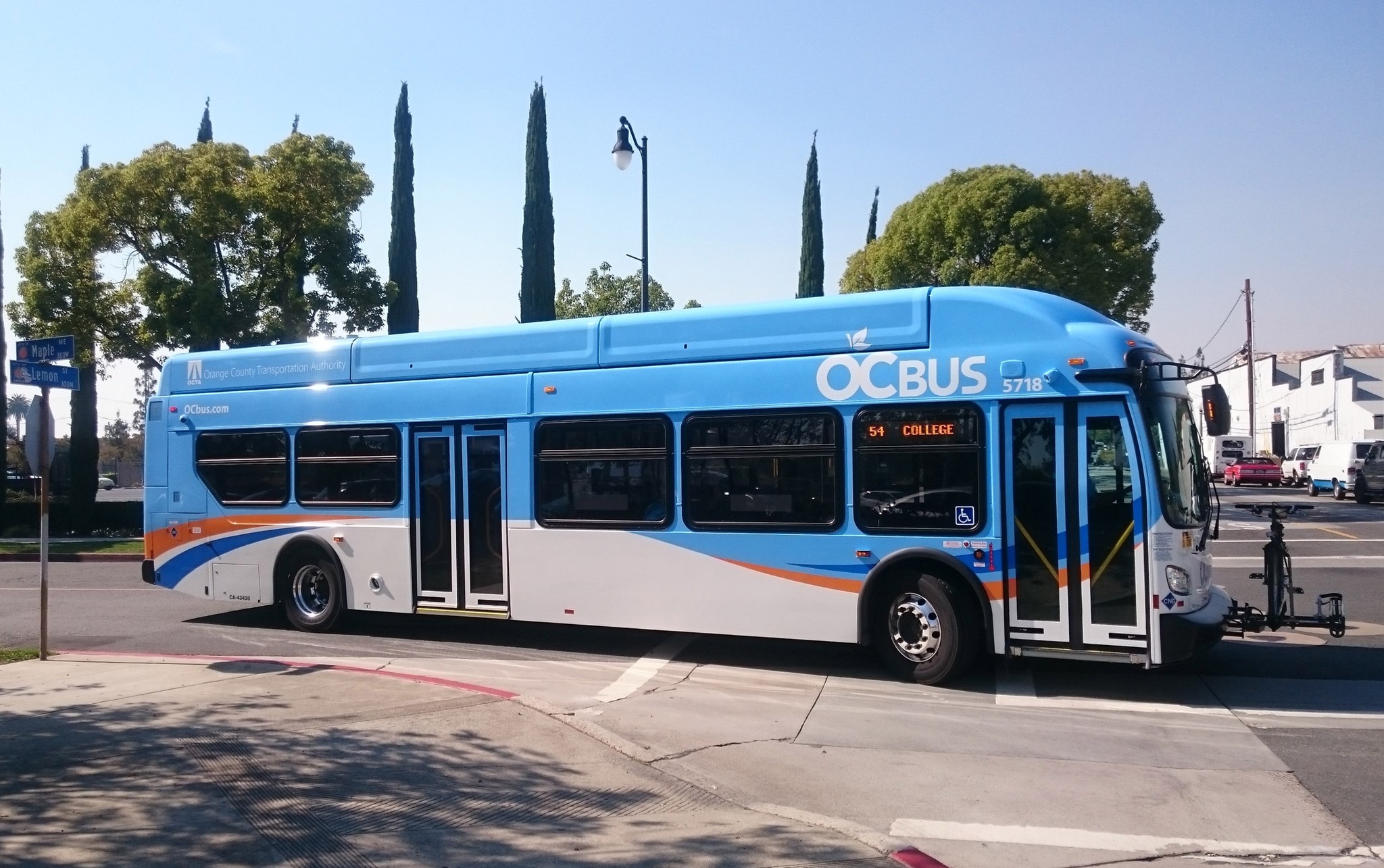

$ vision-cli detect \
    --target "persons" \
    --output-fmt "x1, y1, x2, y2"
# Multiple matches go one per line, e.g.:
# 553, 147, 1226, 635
1088, 440, 1133, 524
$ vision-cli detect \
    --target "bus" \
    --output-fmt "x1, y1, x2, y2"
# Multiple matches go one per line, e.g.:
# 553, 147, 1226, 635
141, 287, 1347, 684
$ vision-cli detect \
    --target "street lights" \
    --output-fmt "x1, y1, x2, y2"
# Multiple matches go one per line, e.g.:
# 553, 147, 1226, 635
610, 116, 649, 312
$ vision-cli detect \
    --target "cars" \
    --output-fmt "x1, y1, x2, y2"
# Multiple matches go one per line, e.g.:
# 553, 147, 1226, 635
98, 474, 115, 491
1092, 450, 1114, 466
1224, 457, 1281, 488
1156, 452, 1162, 463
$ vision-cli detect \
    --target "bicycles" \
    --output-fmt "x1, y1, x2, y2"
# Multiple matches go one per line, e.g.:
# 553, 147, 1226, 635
1236, 504, 1316, 625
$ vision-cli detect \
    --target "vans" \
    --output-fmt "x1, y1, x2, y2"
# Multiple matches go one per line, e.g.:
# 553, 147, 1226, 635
1353, 441, 1384, 505
1091, 441, 1105, 465
1307, 442, 1373, 500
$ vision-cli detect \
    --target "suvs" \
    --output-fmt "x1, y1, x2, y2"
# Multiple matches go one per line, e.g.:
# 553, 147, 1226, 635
1280, 444, 1318, 487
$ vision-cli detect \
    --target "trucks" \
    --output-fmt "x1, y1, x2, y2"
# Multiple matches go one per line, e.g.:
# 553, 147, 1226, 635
1200, 435, 1253, 482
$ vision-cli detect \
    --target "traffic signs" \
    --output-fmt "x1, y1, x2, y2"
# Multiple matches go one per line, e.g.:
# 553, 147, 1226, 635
9, 333, 80, 390
